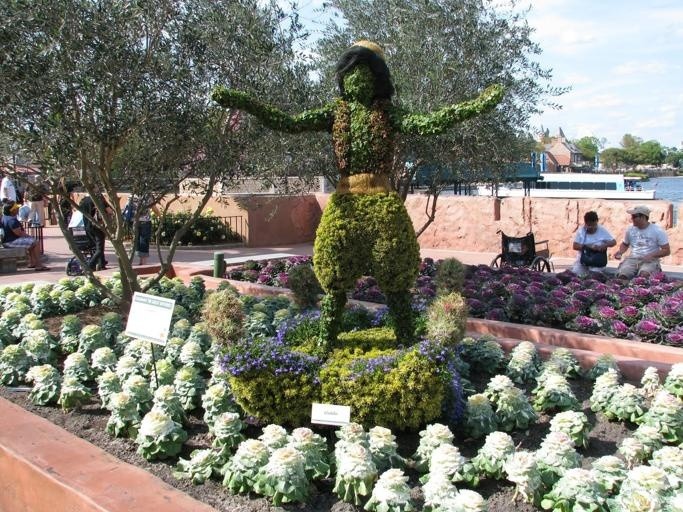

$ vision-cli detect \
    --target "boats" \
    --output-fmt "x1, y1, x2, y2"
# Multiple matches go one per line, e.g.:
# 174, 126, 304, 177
477, 172, 655, 200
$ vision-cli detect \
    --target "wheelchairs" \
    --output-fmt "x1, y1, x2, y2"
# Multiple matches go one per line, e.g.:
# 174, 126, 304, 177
490, 229, 555, 273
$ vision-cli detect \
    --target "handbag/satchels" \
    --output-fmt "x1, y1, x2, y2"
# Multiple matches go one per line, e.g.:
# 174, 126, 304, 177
580, 246, 609, 268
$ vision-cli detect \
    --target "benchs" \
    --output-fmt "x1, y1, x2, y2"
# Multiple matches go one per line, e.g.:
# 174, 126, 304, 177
566, 265, 683, 280
0, 247, 27, 273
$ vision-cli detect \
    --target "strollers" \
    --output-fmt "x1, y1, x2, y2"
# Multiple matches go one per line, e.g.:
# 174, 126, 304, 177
58, 196, 100, 276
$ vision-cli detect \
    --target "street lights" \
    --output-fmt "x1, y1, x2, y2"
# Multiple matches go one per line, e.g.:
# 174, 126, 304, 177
9, 139, 18, 186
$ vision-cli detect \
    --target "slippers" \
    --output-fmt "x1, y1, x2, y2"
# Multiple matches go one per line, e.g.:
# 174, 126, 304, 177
27, 261, 51, 273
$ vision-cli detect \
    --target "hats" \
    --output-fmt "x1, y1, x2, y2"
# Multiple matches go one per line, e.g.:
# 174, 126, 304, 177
10, 201, 21, 211
626, 204, 652, 218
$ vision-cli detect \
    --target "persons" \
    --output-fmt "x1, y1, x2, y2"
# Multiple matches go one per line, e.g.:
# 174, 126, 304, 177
209, 38, 505, 360
77, 178, 114, 271
125, 179, 161, 265
569, 210, 617, 275
0, 165, 75, 272
612, 204, 672, 279
